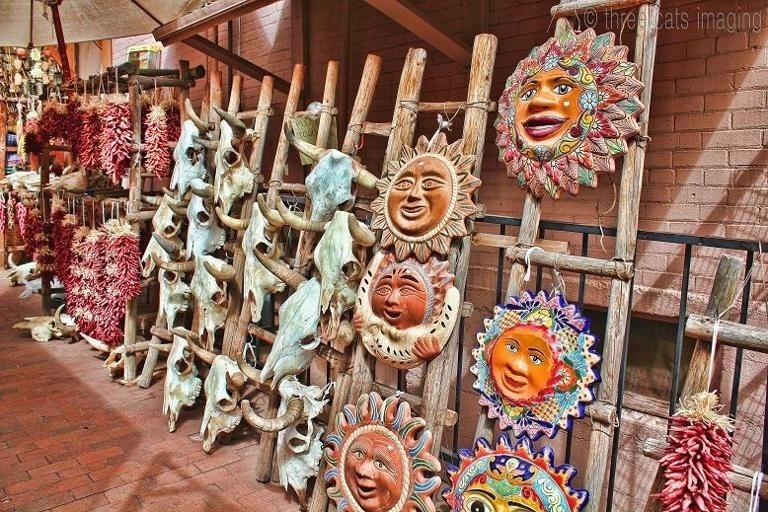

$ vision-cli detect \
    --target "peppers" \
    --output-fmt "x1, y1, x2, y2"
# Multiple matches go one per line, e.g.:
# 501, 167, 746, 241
0, 190, 143, 347
649, 416, 740, 512
23, 93, 181, 187
